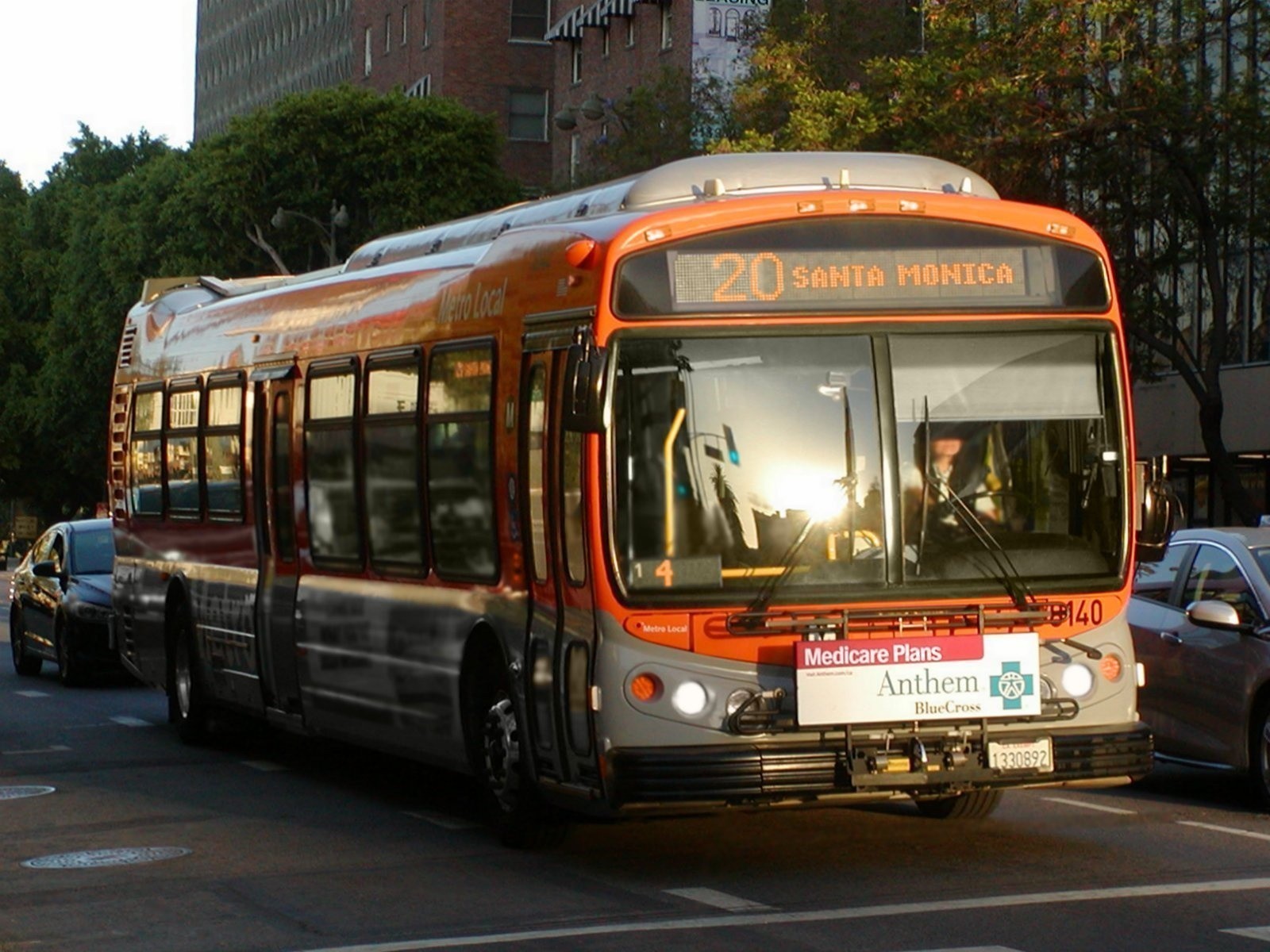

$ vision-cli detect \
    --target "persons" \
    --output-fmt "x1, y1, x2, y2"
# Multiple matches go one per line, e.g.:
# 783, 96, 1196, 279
900, 422, 1004, 527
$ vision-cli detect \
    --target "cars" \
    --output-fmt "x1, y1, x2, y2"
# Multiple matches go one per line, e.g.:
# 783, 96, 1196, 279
1125, 524, 1269, 812
8, 518, 117, 688
308, 478, 495, 560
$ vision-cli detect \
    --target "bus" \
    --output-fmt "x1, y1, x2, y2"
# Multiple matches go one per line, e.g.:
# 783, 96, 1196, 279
107, 148, 1185, 863
